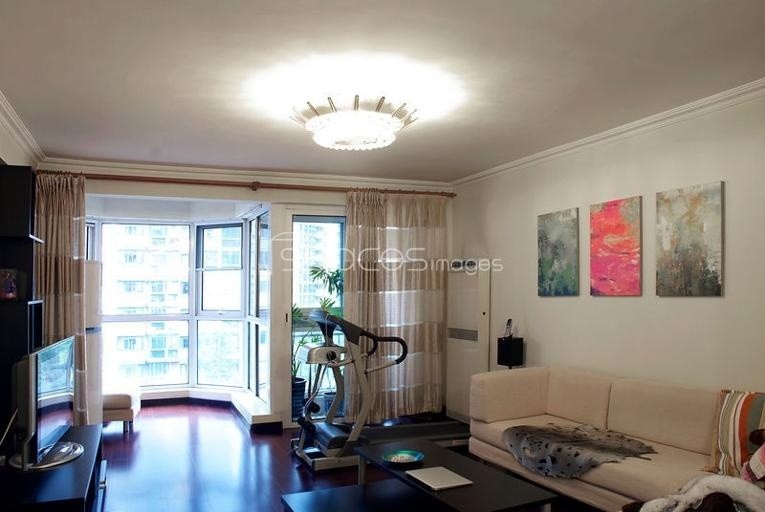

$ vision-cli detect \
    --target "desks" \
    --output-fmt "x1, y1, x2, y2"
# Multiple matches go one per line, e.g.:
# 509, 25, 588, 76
0, 423, 106, 512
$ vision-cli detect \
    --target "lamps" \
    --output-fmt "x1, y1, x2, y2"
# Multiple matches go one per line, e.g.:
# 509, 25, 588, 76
497, 338, 523, 370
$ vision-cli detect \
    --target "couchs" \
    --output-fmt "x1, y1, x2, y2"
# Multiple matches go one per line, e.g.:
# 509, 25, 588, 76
468, 366, 765, 512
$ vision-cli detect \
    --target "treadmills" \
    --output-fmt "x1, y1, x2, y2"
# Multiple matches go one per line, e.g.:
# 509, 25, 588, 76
289, 306, 468, 471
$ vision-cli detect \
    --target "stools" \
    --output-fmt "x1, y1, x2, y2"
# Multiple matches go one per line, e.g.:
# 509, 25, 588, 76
102, 386, 141, 432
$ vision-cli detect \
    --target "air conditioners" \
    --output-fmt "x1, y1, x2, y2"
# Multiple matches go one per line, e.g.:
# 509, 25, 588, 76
444, 265, 492, 425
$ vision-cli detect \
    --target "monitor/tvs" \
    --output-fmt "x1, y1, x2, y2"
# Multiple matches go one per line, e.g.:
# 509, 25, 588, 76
8, 332, 87, 473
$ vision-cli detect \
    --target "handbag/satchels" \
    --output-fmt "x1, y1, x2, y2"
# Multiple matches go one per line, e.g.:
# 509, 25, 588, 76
497, 319, 524, 366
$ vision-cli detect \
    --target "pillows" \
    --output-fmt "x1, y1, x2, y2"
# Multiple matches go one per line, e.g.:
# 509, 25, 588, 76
701, 388, 765, 479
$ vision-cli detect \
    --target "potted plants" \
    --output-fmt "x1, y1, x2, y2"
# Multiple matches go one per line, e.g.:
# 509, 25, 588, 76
292, 260, 343, 416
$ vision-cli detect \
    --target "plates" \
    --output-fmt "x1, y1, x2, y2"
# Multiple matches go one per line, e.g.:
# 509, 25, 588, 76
382, 450, 425, 464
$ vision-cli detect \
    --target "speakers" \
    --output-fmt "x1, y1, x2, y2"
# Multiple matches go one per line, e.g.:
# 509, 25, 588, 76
497, 336, 524, 366
